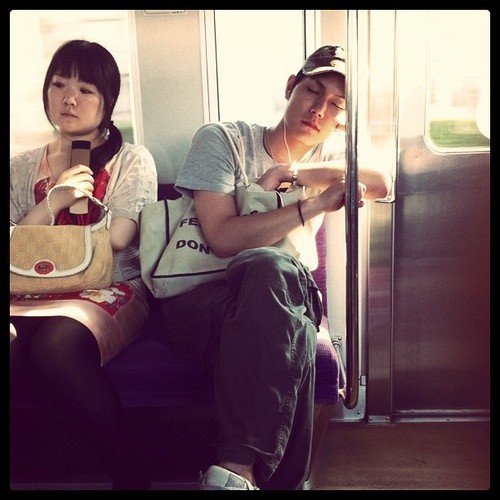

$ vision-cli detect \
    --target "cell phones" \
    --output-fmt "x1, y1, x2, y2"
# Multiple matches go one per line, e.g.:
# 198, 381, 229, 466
68, 140, 91, 215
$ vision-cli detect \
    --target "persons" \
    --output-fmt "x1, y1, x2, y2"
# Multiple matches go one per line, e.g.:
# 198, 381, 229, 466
9, 40, 157, 491
160, 45, 394, 491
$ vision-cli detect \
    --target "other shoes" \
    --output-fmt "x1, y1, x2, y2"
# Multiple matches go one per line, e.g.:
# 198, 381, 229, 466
199, 464, 260, 490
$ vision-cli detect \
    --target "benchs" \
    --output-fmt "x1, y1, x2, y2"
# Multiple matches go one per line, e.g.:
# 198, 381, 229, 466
158, 183, 338, 407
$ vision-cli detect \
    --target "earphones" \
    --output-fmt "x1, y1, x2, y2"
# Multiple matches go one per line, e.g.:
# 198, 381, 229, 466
287, 90, 292, 97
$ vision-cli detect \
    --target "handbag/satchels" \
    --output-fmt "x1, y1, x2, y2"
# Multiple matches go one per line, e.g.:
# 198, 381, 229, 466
139, 120, 319, 298
11, 184, 113, 294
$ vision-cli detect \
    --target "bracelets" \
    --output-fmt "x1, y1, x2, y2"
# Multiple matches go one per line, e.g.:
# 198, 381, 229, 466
297, 199, 306, 227
291, 168, 298, 186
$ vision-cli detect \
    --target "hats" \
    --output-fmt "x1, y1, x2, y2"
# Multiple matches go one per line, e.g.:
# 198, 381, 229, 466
297, 45, 345, 76
